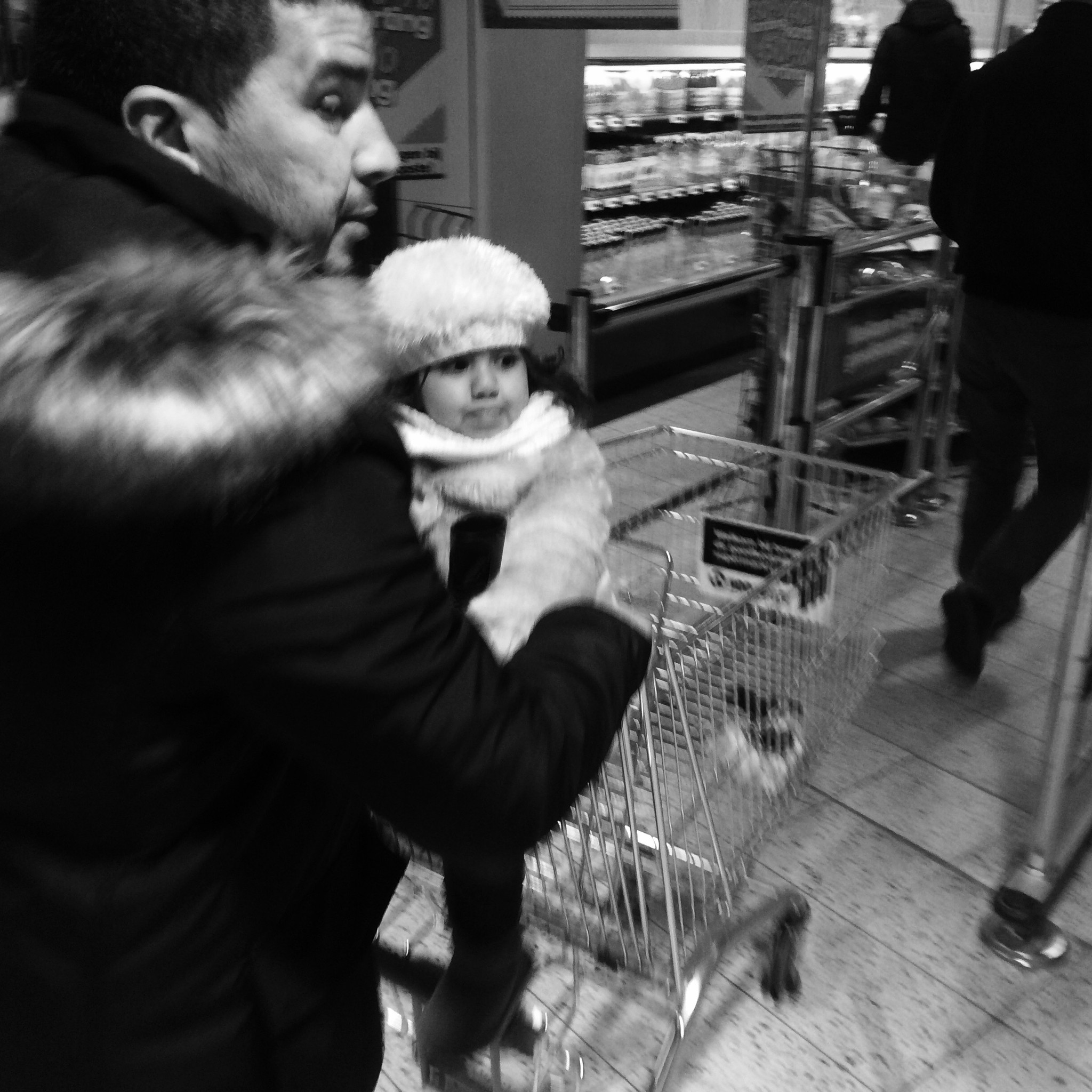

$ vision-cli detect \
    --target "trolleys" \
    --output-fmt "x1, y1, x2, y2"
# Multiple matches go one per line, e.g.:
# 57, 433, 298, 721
368, 421, 916, 1092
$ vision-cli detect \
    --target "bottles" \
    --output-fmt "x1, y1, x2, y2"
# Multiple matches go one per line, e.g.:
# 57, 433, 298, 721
583, 64, 758, 300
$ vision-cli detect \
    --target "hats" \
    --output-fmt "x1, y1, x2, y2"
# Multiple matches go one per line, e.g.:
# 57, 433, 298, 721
367, 235, 552, 382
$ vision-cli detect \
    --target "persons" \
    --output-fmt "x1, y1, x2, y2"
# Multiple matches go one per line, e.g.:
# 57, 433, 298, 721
0, 0, 653, 1092
848, 0, 1092, 682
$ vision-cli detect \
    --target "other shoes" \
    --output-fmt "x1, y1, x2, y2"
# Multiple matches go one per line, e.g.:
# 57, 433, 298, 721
989, 590, 1021, 643
414, 946, 528, 1069
939, 587, 985, 682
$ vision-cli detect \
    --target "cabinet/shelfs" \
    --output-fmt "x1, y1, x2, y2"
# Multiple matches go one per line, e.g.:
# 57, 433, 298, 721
738, 163, 956, 529
